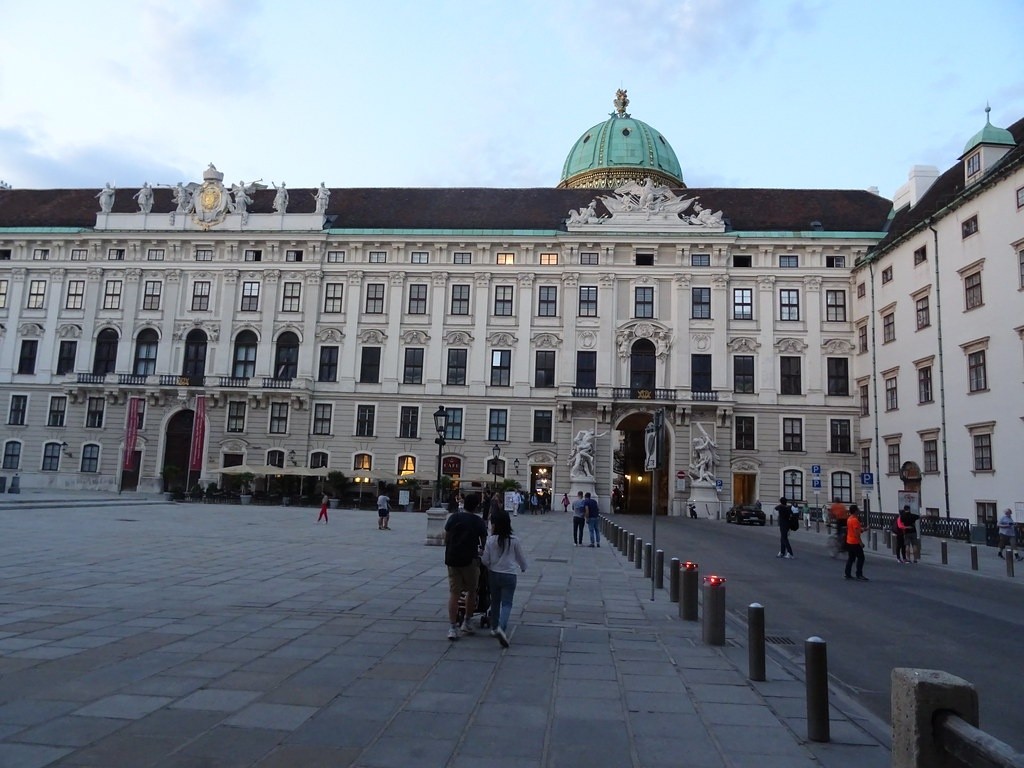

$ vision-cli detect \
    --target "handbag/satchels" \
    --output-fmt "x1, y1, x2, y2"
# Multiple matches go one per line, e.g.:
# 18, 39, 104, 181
790, 514, 799, 532
889, 517, 901, 535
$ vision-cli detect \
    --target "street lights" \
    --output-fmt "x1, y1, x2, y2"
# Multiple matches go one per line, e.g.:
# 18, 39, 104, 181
423, 404, 449, 546
491, 443, 501, 492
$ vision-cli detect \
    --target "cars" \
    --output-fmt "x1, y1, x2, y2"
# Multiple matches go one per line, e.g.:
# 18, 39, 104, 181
725, 505, 767, 526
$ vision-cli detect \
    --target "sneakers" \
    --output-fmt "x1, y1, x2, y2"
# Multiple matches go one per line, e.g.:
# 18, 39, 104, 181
460, 621, 479, 634
447, 630, 459, 640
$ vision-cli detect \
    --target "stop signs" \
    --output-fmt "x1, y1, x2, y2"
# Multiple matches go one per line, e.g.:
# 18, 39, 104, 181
677, 471, 686, 479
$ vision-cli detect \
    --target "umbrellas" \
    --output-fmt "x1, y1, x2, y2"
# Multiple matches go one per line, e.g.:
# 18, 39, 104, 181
206, 463, 505, 504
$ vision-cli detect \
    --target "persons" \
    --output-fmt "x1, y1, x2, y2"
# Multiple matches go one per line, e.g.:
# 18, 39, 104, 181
574, 428, 608, 477
895, 505, 921, 563
480, 508, 528, 648
455, 488, 570, 529
94, 180, 116, 213
271, 181, 289, 214
314, 181, 331, 214
376, 490, 392, 530
845, 505, 870, 581
801, 503, 811, 528
774, 497, 794, 559
132, 181, 155, 214
572, 491, 600, 547
226, 180, 255, 213
821, 505, 829, 525
167, 182, 195, 214
692, 433, 712, 481
791, 502, 800, 519
831, 497, 850, 536
313, 490, 328, 524
997, 508, 1024, 562
444, 494, 488, 638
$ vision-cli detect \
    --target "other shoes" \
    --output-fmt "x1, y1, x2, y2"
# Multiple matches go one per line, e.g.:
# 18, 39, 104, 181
856, 575, 869, 582
588, 544, 595, 547
897, 560, 903, 564
596, 544, 600, 547
776, 551, 784, 558
998, 554, 1005, 560
574, 542, 577, 546
578, 543, 583, 546
490, 629, 496, 637
1015, 557, 1024, 562
784, 553, 794, 560
903, 559, 911, 563
496, 627, 510, 648
844, 575, 855, 580
914, 561, 918, 563
